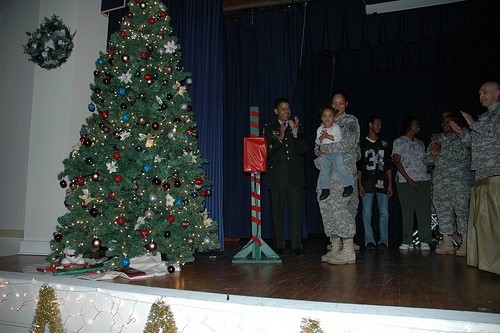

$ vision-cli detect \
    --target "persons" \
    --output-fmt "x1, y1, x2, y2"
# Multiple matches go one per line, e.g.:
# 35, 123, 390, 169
315, 104, 355, 200
262, 97, 308, 258
313, 92, 360, 264
390, 113, 433, 250
449, 82, 500, 277
422, 112, 474, 257
354, 115, 393, 250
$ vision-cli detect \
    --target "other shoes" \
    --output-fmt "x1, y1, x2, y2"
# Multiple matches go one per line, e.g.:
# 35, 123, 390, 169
367, 241, 375, 248
379, 242, 387, 249
353, 242, 360, 249
420, 241, 430, 250
319, 188, 330, 199
399, 243, 410, 249
342, 185, 353, 196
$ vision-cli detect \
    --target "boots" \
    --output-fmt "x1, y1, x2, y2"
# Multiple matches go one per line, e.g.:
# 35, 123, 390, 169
435, 234, 454, 253
456, 235, 467, 256
321, 237, 343, 261
328, 237, 356, 264
